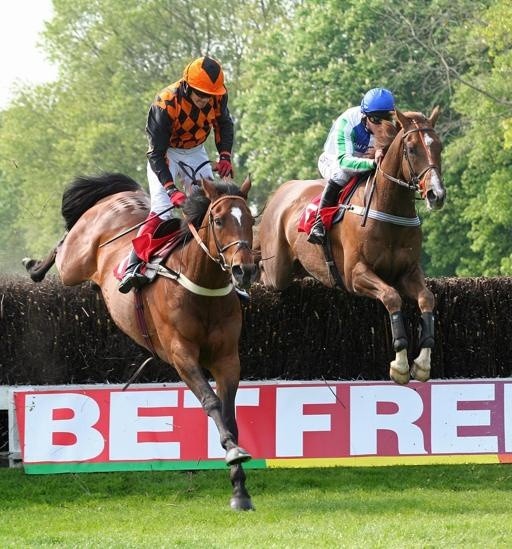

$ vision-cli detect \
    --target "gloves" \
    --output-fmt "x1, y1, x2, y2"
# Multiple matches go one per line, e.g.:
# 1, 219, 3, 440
212, 150, 234, 179
164, 181, 187, 208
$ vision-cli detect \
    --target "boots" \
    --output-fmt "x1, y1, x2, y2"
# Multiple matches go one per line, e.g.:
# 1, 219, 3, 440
307, 179, 344, 244
118, 212, 165, 293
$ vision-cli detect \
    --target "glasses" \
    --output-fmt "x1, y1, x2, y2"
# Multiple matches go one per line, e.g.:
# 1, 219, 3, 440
364, 112, 381, 124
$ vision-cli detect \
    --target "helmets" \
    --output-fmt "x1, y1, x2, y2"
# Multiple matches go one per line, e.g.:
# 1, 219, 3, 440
182, 56, 227, 96
360, 88, 395, 114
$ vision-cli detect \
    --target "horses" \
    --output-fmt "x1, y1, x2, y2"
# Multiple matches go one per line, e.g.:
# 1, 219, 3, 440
249, 102, 446, 386
20, 170, 256, 512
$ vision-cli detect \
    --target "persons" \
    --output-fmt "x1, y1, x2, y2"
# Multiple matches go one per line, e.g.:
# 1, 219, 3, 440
116, 56, 257, 304
303, 87, 396, 245
